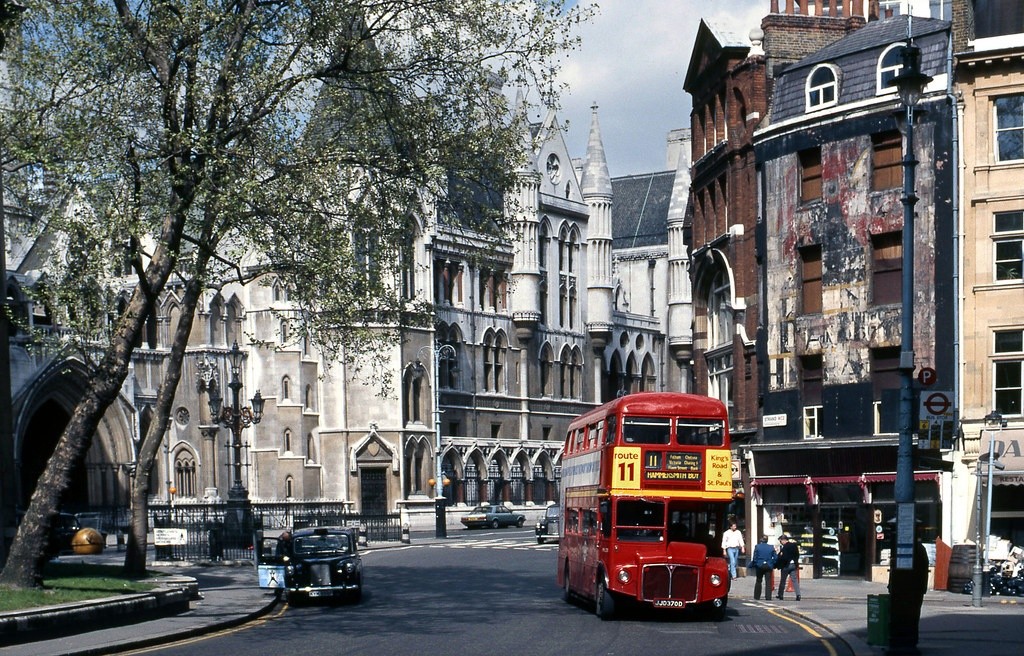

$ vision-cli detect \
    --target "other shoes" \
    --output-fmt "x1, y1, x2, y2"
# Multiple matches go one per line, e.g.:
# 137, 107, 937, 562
732, 577, 737, 581
776, 596, 783, 600
796, 595, 801, 601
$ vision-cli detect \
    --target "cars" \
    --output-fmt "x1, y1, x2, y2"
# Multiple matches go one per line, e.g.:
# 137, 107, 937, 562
257, 527, 364, 606
461, 504, 526, 528
534, 503, 560, 544
50, 513, 108, 554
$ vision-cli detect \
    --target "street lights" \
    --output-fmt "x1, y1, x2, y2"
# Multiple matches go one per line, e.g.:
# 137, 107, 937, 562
162, 417, 177, 523
970, 459, 1005, 607
979, 409, 1007, 597
209, 338, 266, 550
418, 338, 458, 537
889, 2, 933, 655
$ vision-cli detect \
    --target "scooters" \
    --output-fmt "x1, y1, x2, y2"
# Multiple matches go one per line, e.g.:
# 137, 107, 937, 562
962, 564, 1024, 597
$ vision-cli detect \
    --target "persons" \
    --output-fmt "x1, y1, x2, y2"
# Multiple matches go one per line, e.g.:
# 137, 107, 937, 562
775, 534, 802, 601
720, 520, 747, 583
747, 534, 782, 600
915, 529, 931, 643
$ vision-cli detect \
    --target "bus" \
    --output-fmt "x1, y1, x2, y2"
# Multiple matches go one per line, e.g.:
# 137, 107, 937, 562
553, 392, 732, 621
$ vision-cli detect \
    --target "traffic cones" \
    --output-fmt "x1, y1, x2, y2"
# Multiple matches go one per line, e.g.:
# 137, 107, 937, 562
785, 564, 799, 592
770, 569, 775, 590
